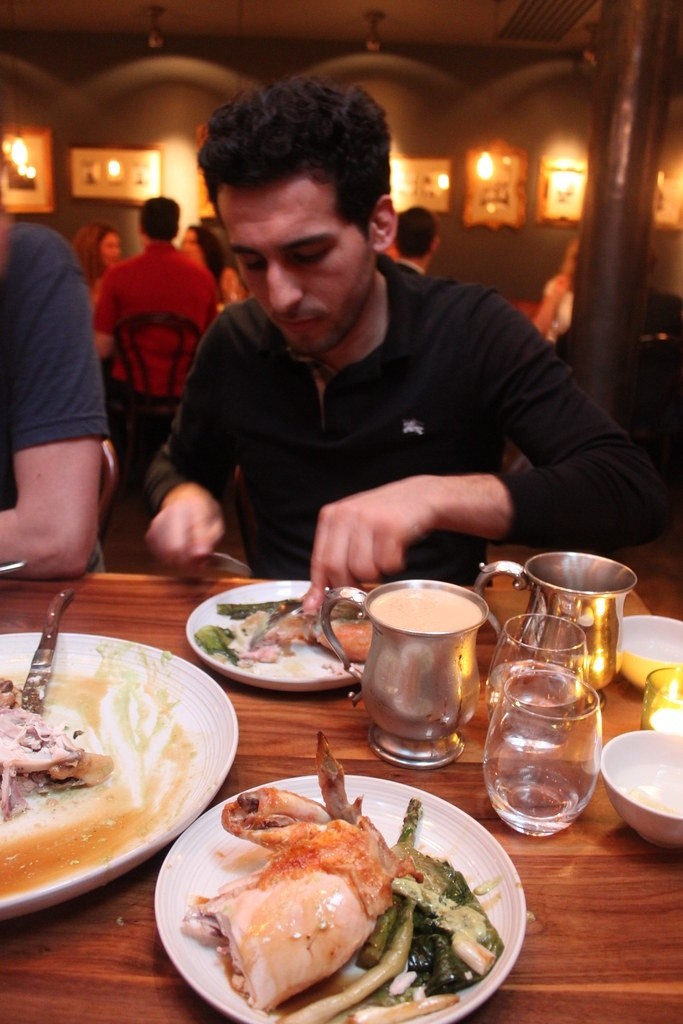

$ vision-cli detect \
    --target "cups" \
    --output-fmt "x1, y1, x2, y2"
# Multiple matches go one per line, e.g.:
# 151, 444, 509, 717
320, 579, 490, 770
473, 552, 637, 712
482, 670, 603, 838
640, 668, 681, 732
485, 613, 590, 719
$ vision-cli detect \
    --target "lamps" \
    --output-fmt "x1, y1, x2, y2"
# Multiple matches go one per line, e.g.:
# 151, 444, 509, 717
145, 6, 166, 49
358, 12, 386, 53
581, 21, 601, 68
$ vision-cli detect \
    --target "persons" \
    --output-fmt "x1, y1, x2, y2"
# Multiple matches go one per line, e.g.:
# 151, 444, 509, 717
143, 74, 674, 616
73, 197, 243, 457
391, 205, 439, 276
0, 221, 117, 578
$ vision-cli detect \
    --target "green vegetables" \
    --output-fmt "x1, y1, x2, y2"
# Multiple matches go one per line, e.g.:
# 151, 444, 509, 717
192, 624, 241, 668
381, 853, 503, 1006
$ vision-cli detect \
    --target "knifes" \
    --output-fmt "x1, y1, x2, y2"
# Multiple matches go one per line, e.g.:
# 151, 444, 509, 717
213, 553, 252, 577
21, 588, 75, 717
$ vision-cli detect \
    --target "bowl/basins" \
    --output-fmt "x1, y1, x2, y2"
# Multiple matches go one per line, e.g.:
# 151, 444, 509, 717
600, 730, 683, 849
620, 615, 683, 694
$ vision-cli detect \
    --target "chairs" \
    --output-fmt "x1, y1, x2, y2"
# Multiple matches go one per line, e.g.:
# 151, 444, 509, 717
107, 314, 201, 498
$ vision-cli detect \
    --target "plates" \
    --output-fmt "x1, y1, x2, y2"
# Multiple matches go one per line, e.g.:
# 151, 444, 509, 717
186, 580, 366, 690
0, 632, 239, 921
154, 774, 527, 1024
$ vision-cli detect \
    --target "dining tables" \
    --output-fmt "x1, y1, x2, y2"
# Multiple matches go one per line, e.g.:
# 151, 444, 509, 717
1, 564, 683, 1024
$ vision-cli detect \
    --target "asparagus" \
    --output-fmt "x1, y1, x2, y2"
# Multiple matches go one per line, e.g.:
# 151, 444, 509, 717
217, 599, 303, 619
356, 799, 424, 965
